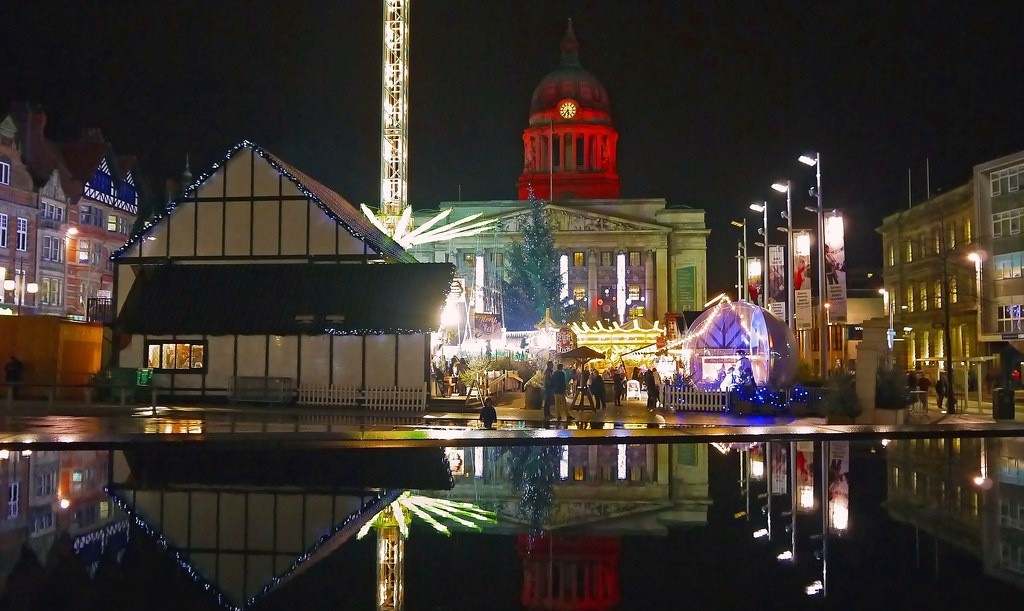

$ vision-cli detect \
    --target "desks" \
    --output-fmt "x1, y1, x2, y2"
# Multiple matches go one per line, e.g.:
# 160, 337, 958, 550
910, 391, 928, 417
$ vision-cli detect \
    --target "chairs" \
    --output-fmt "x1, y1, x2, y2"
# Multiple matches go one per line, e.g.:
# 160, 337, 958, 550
625, 379, 641, 402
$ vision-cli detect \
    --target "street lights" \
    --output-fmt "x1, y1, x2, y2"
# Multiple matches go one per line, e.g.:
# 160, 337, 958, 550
775, 442, 798, 562
731, 218, 750, 302
799, 151, 830, 383
750, 443, 770, 542
803, 441, 829, 596
770, 178, 796, 337
749, 200, 770, 313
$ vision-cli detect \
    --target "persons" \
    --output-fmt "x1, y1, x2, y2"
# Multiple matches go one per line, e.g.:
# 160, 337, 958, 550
562, 364, 664, 402
645, 370, 657, 412
916, 369, 932, 413
769, 266, 784, 303
748, 281, 760, 307
905, 368, 918, 412
520, 334, 529, 360
590, 369, 607, 410
824, 243, 840, 284
935, 374, 946, 410
430, 349, 473, 399
613, 368, 624, 407
479, 397, 497, 428
794, 256, 809, 290
549, 363, 577, 420
982, 361, 1023, 397
543, 360, 556, 418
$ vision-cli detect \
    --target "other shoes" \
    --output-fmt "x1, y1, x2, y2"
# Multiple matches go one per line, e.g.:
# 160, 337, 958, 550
602, 406, 607, 409
615, 404, 623, 406
544, 414, 556, 419
557, 416, 562, 419
567, 416, 575, 420
595, 407, 600, 409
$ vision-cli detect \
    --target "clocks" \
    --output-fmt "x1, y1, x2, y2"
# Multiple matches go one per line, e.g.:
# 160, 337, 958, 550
559, 102, 577, 120
